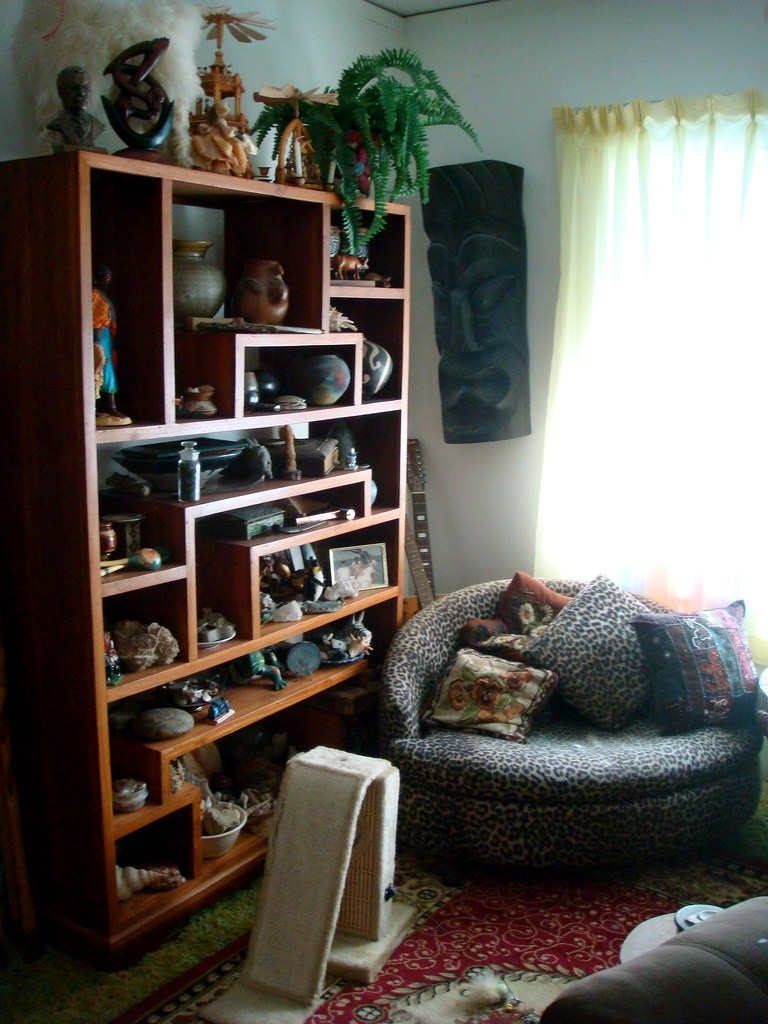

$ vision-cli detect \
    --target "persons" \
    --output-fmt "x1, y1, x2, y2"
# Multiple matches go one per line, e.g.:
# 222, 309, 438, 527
338, 551, 378, 587
46, 63, 104, 144
92, 256, 124, 417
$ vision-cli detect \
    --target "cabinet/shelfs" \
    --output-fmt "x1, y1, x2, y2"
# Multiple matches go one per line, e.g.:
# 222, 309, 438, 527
1, 148, 412, 967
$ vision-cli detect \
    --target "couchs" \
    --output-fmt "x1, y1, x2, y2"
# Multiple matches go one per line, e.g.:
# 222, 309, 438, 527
379, 576, 768, 882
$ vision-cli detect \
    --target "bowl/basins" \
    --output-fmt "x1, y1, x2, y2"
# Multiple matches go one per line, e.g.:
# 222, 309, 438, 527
158, 678, 225, 712
201, 801, 248, 860
246, 804, 274, 828
109, 438, 242, 492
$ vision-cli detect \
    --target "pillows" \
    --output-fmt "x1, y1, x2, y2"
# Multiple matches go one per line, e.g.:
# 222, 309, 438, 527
421, 570, 759, 743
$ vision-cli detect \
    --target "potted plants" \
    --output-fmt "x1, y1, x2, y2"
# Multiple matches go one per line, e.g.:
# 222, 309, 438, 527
250, 48, 485, 254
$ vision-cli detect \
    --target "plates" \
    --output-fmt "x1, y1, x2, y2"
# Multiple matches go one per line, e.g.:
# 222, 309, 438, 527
321, 653, 365, 663
674, 904, 724, 930
198, 631, 237, 648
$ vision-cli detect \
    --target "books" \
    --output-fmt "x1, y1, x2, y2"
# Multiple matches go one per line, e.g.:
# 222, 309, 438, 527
261, 438, 339, 477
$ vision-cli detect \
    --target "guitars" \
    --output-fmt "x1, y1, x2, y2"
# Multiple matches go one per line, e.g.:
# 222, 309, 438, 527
403, 438, 450, 623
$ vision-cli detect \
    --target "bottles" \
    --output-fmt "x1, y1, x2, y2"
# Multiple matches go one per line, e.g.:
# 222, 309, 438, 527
177, 440, 201, 502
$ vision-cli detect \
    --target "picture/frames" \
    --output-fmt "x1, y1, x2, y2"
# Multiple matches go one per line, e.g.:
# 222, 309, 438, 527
329, 542, 389, 592
